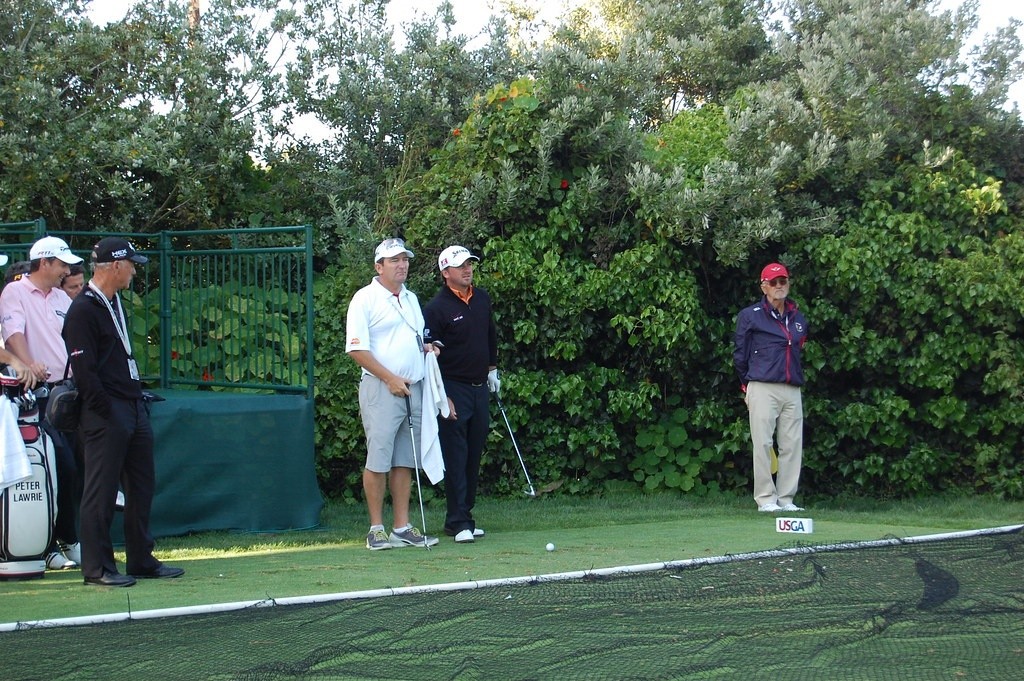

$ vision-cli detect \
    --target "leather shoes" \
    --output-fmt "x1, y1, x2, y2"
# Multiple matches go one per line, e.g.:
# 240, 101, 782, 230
126, 559, 186, 580
84, 568, 137, 588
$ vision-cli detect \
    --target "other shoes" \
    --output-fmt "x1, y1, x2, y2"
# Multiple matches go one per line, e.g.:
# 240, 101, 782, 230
453, 529, 473, 544
472, 521, 484, 537
781, 503, 804, 510
758, 502, 783, 511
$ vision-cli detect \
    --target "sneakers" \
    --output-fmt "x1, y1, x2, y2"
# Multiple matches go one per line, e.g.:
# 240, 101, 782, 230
46, 547, 76, 570
390, 524, 439, 548
54, 538, 82, 565
365, 528, 391, 550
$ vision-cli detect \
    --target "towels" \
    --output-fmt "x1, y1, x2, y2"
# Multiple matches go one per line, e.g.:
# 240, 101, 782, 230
0, 394, 33, 496
420, 351, 450, 486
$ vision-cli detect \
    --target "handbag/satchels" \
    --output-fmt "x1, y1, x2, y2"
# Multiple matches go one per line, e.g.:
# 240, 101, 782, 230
45, 380, 80, 431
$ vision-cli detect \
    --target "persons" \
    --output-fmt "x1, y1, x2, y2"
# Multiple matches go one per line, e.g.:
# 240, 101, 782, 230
60, 237, 185, 586
0, 236, 87, 570
345, 238, 440, 551
421, 246, 501, 543
734, 263, 808, 511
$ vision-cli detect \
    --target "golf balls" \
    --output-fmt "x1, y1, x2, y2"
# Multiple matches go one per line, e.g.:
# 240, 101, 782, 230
546, 542, 555, 552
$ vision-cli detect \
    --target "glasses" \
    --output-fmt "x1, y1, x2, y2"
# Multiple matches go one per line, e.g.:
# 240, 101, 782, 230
765, 277, 786, 286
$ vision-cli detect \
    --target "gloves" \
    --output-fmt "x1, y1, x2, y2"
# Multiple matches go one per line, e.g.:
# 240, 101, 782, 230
488, 368, 501, 393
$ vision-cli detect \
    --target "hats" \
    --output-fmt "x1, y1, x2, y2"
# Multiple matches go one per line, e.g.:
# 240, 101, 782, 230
91, 236, 149, 264
30, 235, 84, 265
762, 263, 789, 280
438, 244, 480, 272
0, 254, 8, 266
374, 237, 415, 263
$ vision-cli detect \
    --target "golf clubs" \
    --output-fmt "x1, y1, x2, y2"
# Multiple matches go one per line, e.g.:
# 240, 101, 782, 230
404, 382, 432, 552
493, 391, 536, 496
1, 386, 49, 413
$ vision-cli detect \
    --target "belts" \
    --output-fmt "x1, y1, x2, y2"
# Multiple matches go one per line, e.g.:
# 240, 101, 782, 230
470, 381, 483, 387
